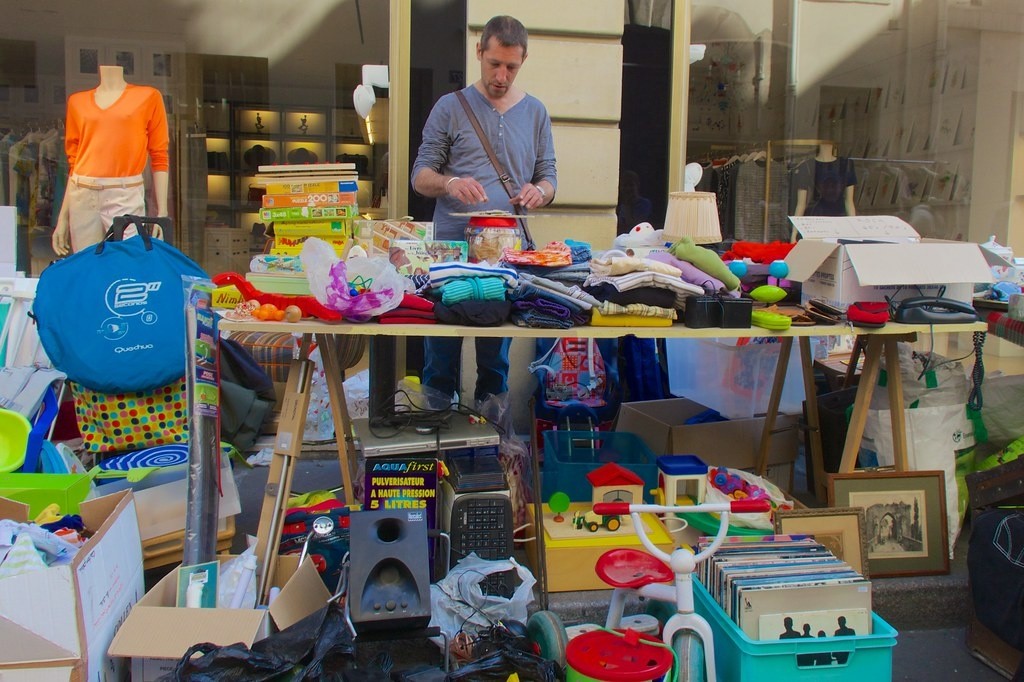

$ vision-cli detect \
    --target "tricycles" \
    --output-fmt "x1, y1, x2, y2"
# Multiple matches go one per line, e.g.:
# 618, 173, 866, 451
527, 498, 774, 682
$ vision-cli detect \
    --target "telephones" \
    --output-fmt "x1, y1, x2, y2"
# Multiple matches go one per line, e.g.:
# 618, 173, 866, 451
895, 297, 979, 324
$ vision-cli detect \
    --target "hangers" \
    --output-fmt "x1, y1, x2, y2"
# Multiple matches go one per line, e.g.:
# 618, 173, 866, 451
0, 118, 65, 144
704, 144, 773, 170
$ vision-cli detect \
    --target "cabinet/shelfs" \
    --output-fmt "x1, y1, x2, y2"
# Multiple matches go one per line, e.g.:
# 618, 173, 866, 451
205, 228, 250, 276
203, 99, 389, 228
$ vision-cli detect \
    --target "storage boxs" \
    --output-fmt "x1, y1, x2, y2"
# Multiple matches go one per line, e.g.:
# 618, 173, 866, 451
1, 183, 1024, 682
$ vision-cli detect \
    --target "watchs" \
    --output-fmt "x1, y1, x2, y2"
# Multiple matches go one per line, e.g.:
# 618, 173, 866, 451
535, 185, 547, 199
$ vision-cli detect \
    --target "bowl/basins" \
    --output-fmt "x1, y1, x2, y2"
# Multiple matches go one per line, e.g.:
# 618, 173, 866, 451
0, 408, 33, 473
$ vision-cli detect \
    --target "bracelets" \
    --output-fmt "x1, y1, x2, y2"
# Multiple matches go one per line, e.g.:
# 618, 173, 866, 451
445, 177, 460, 194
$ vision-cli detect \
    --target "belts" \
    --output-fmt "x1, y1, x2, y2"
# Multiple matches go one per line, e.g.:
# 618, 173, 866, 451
71, 181, 143, 190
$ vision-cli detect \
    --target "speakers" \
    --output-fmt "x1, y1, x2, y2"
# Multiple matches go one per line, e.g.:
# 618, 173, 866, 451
349, 507, 431, 631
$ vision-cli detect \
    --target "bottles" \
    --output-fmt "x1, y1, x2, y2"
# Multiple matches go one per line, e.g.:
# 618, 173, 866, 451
464, 217, 521, 264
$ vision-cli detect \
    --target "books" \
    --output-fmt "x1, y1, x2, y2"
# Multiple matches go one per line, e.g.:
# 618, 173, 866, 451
699, 534, 872, 642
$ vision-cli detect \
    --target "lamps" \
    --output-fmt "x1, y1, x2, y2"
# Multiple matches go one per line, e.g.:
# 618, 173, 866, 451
665, 190, 722, 247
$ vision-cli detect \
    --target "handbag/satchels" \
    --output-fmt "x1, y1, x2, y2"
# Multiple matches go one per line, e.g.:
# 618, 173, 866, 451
427, 551, 537, 648
248, 186, 267, 201
69, 378, 189, 454
31, 213, 212, 391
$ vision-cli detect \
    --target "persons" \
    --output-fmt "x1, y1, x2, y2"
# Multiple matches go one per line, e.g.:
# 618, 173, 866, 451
411, 15, 557, 411
51, 65, 169, 256
789, 144, 857, 244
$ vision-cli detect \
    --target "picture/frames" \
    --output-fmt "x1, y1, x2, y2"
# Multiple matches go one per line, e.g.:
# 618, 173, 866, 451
826, 469, 951, 576
685, 80, 752, 138
773, 507, 870, 581
801, 59, 980, 204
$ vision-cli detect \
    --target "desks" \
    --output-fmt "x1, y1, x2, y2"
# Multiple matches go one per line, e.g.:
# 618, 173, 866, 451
216, 316, 989, 575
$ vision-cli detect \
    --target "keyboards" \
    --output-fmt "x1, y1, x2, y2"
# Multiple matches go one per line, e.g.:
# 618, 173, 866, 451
450, 493, 515, 601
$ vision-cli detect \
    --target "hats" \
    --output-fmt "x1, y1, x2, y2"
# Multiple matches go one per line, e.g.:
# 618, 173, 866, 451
287, 148, 317, 163
244, 145, 275, 166
